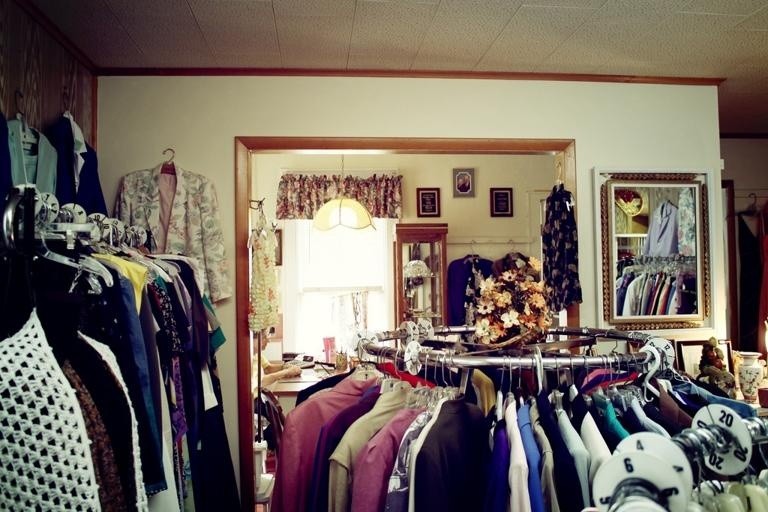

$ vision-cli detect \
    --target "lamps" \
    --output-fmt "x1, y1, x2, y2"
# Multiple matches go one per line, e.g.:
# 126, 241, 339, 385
312, 155, 377, 233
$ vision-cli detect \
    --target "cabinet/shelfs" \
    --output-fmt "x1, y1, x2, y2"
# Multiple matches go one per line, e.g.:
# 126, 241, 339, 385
394, 222, 448, 327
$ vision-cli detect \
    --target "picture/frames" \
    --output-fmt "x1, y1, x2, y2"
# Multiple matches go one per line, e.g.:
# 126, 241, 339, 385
592, 166, 716, 331
453, 168, 476, 198
252, 228, 283, 266
416, 187, 440, 217
489, 187, 513, 217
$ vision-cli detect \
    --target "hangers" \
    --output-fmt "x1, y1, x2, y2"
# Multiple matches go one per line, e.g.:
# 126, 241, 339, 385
464, 238, 520, 260
554, 162, 564, 185
726, 193, 764, 218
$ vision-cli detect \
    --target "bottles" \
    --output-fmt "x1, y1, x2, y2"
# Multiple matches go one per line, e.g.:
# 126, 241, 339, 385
738, 351, 762, 404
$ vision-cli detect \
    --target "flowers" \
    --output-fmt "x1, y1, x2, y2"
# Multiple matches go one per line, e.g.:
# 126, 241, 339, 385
465, 253, 557, 350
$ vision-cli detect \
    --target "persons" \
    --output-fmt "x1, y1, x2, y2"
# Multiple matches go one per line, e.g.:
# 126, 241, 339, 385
251, 327, 302, 454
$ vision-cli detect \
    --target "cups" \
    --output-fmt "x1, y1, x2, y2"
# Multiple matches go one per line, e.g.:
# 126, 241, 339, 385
336, 353, 347, 371
